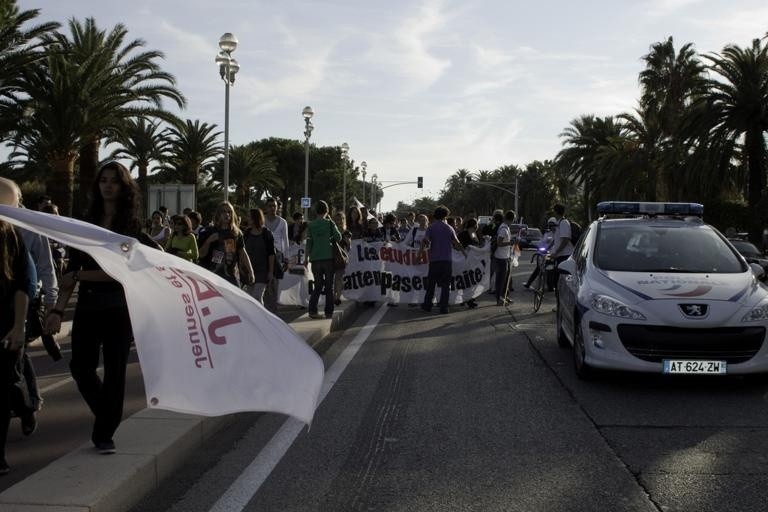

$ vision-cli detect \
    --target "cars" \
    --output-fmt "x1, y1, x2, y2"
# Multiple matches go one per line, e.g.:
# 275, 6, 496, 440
722, 231, 768, 282
477, 215, 543, 248
556, 199, 768, 382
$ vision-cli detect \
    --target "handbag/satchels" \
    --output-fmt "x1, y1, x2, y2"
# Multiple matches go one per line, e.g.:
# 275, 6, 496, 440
332, 240, 349, 270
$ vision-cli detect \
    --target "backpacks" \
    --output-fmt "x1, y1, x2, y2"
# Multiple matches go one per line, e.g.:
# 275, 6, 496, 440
558, 217, 582, 247
262, 227, 285, 279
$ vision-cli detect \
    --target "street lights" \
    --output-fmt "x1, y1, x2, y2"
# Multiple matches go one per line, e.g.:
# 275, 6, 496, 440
340, 142, 349, 212
301, 106, 314, 222
215, 30, 240, 202
360, 160, 367, 206
371, 173, 382, 214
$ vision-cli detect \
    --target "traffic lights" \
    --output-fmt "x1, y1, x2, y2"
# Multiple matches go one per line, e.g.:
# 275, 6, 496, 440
418, 176, 423, 188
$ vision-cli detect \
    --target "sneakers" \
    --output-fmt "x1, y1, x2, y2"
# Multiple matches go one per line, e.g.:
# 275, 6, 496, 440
20, 411, 37, 437
91, 417, 102, 444
496, 295, 512, 306
420, 303, 450, 314
94, 436, 117, 454
309, 311, 332, 319
0, 457, 11, 474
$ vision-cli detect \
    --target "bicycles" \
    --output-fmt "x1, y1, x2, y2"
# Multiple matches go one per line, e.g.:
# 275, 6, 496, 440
529, 252, 553, 312
